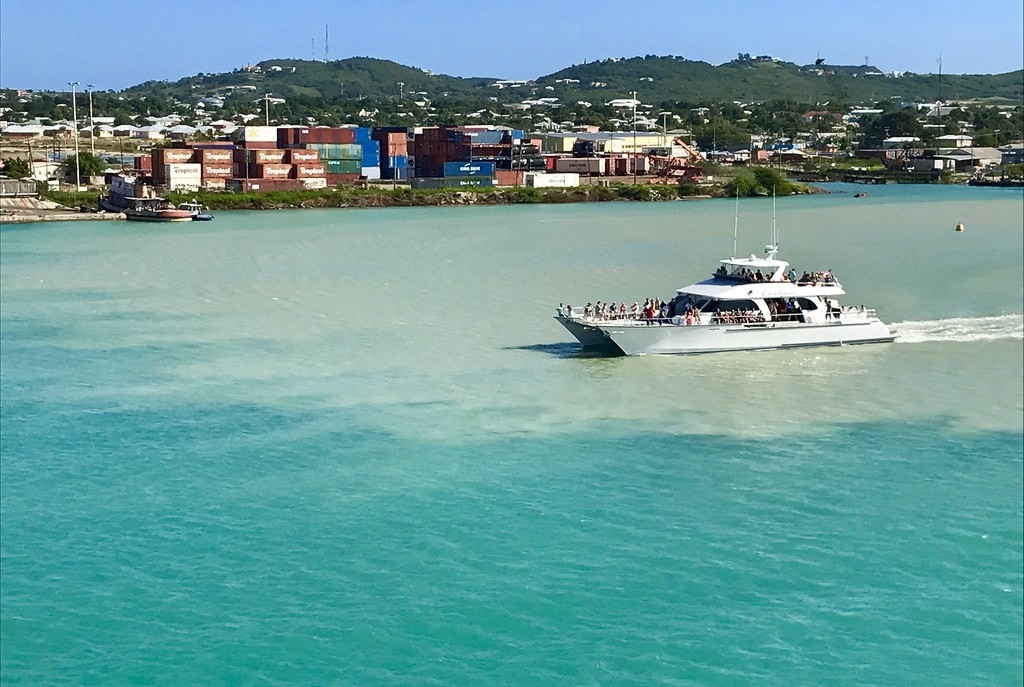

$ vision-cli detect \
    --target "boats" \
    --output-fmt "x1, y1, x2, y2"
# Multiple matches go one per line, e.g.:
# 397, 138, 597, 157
100, 173, 192, 222
178, 199, 212, 220
552, 185, 901, 356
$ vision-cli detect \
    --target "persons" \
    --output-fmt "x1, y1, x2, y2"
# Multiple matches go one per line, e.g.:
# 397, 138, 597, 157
716, 266, 727, 275
610, 302, 619, 320
685, 299, 702, 325
567, 305, 575, 322
647, 297, 676, 326
602, 302, 607, 319
584, 302, 593, 318
596, 301, 604, 317
826, 301, 831, 319
557, 303, 568, 318
629, 302, 639, 319
738, 268, 774, 282
620, 302, 628, 319
769, 297, 805, 323
640, 298, 650, 318
842, 304, 866, 313
783, 268, 837, 287
715, 307, 765, 325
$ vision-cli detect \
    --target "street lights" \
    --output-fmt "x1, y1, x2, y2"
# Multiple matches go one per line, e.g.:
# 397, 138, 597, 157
87, 83, 95, 175
68, 81, 80, 192
265, 93, 272, 126
